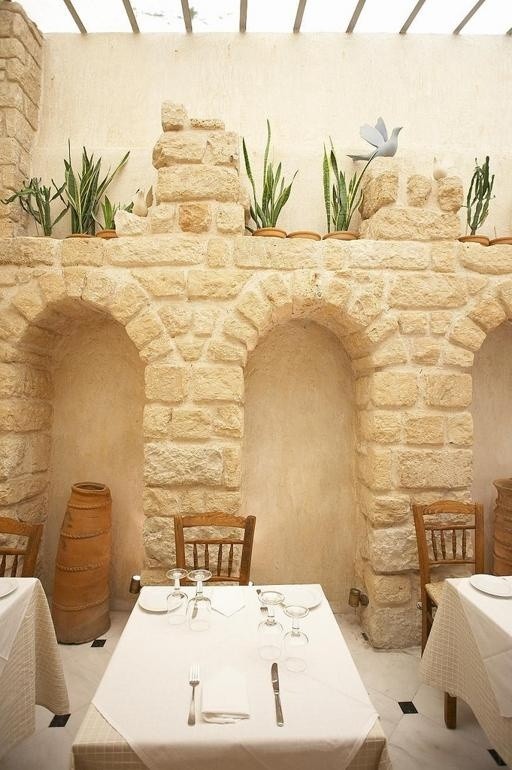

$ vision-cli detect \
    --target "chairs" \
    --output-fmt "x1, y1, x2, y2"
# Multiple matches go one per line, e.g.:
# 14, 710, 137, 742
173, 512, 256, 586
412, 500, 484, 658
0, 517, 43, 577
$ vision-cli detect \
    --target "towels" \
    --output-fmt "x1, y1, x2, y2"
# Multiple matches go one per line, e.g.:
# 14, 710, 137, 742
201, 686, 250, 724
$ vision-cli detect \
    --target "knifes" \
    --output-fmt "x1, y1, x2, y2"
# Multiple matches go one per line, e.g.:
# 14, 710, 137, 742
269, 662, 286, 727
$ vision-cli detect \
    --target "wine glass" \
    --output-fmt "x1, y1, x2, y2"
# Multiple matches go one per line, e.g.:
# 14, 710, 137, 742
165, 567, 211, 626
256, 589, 310, 672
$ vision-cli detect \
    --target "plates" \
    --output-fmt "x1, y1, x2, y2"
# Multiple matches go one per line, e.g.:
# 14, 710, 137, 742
140, 590, 181, 612
281, 585, 324, 609
0, 577, 17, 599
470, 573, 511, 601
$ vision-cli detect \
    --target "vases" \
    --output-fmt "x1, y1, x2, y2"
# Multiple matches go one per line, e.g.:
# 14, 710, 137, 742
287, 231, 321, 241
489, 237, 512, 245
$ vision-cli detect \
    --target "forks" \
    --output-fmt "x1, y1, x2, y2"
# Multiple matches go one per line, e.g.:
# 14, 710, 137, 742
184, 663, 200, 729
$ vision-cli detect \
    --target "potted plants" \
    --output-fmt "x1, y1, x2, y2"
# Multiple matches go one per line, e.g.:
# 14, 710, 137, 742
64, 138, 130, 238
322, 136, 364, 240
243, 120, 299, 238
458, 156, 495, 246
96, 195, 134, 238
